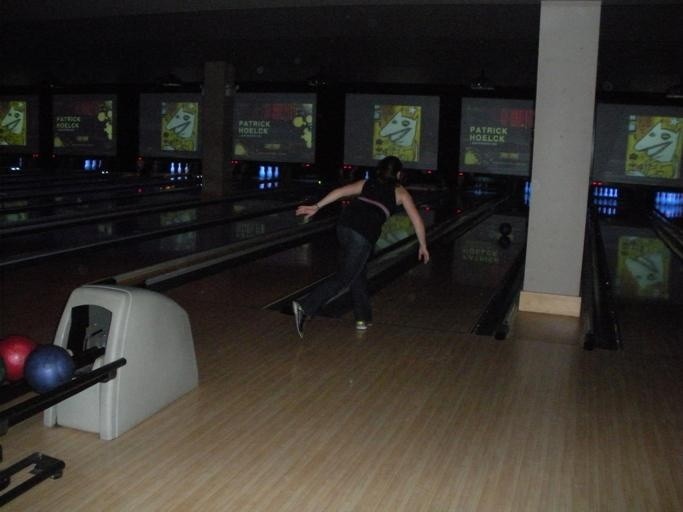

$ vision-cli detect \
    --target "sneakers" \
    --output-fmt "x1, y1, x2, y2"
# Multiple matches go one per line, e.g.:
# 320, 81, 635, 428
293, 301, 305, 338
356, 321, 373, 330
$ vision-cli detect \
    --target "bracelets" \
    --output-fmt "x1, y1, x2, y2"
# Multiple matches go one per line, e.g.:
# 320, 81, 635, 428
315, 203, 321, 210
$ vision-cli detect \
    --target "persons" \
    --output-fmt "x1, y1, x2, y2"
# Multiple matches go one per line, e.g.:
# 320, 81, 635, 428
291, 155, 430, 340
